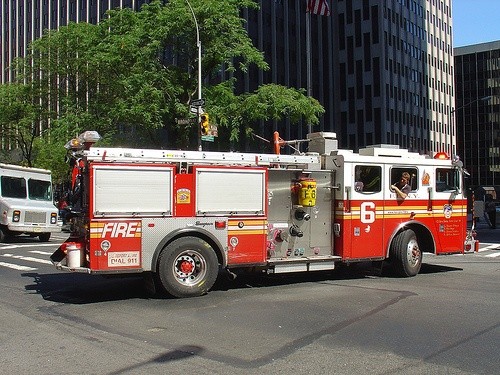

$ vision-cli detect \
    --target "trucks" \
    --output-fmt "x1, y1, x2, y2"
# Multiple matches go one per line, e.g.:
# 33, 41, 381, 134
0, 162, 62, 243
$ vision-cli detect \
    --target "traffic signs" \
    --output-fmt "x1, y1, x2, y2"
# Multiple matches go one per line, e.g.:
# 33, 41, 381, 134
192, 98, 206, 107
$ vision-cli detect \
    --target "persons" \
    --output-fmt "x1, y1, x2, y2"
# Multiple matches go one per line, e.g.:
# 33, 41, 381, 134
486, 198, 496, 226
392, 172, 411, 199
58, 198, 67, 215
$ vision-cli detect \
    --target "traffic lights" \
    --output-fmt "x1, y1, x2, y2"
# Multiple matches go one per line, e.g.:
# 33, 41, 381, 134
200, 113, 209, 135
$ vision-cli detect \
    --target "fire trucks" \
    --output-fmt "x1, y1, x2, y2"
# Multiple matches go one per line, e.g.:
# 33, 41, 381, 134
54, 130, 480, 299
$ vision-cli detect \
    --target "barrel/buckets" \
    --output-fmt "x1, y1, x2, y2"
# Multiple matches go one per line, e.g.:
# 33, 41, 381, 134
298, 178, 317, 206
66, 244, 86, 267
